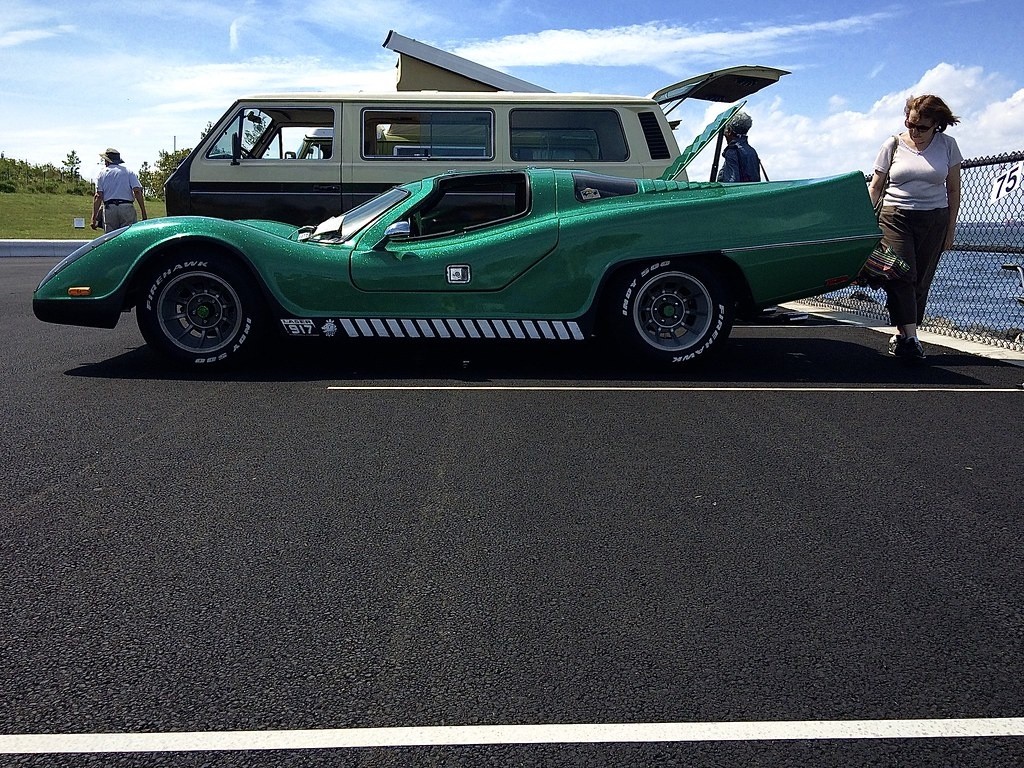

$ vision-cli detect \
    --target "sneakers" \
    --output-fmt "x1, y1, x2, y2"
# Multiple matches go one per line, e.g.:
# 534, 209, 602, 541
887, 333, 903, 357
901, 333, 927, 359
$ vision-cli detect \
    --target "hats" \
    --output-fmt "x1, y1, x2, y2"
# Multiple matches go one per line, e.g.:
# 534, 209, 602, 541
99, 148, 124, 164
725, 110, 752, 136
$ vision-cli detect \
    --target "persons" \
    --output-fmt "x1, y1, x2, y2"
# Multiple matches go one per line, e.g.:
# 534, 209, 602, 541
717, 113, 761, 182
89, 147, 147, 234
869, 95, 963, 359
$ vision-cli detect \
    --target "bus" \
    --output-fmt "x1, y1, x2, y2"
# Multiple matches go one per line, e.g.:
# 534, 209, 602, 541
160, 63, 793, 232
294, 27, 684, 164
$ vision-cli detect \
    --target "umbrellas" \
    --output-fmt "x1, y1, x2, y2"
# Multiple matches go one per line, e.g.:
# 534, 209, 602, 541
854, 245, 909, 289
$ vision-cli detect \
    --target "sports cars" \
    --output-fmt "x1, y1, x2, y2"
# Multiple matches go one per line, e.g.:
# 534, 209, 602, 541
26, 167, 887, 382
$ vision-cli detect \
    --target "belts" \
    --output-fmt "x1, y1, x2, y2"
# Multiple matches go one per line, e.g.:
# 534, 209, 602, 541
104, 199, 129, 204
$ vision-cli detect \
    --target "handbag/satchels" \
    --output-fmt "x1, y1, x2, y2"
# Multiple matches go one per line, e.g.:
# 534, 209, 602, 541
872, 135, 899, 219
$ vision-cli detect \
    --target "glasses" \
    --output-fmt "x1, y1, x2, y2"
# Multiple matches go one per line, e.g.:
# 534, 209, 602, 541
904, 116, 934, 133
